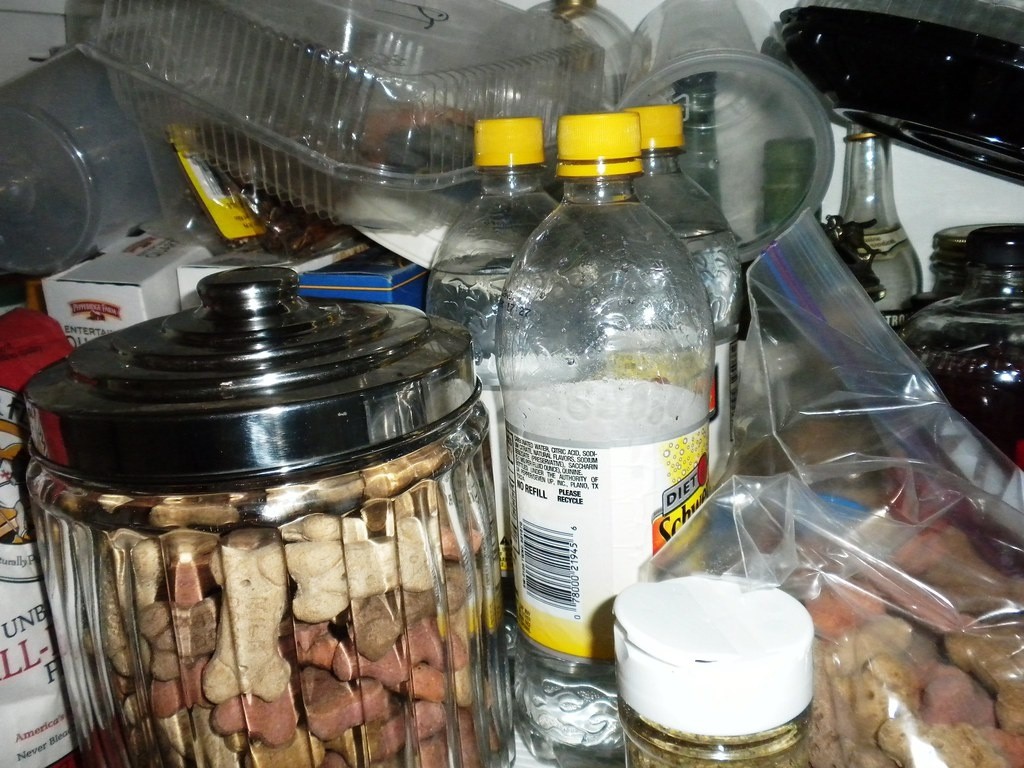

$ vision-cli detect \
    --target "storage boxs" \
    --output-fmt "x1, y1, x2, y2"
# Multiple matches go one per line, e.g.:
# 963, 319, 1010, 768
298, 246, 427, 308
176, 243, 366, 312
780, 5, 1024, 294
41, 230, 212, 347
74, 0, 604, 233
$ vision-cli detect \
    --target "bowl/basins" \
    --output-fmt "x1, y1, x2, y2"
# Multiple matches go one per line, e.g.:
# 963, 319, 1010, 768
2, 50, 197, 272
74, 0, 606, 232
624, 1, 835, 262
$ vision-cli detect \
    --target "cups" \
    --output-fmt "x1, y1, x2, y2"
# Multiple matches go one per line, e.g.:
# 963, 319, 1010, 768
613, 576, 814, 766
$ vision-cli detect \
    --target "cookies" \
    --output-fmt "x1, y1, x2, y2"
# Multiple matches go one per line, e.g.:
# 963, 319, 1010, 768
805, 529, 1024, 768
30, 442, 506, 768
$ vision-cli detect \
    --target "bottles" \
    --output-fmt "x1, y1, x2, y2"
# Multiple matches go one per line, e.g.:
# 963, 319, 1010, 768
839, 123, 923, 327
24, 265, 523, 766
620, 101, 740, 458
895, 225, 1023, 470
428, 117, 595, 349
757, 138, 822, 236
893, 226, 981, 328
494, 112, 714, 744
670, 68, 718, 216
